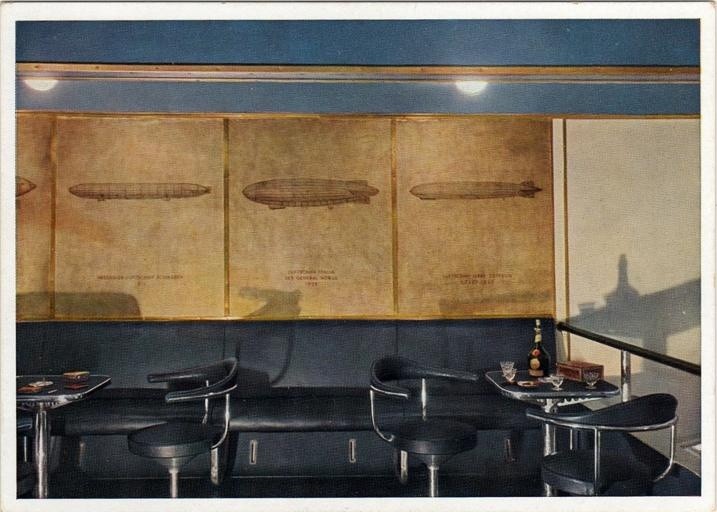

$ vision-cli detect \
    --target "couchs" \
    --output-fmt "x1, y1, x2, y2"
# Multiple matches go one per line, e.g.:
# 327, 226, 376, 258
17, 319, 557, 487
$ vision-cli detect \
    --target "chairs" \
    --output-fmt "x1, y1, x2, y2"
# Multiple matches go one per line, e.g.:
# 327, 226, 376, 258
369, 352, 482, 498
124, 358, 238, 496
523, 392, 679, 496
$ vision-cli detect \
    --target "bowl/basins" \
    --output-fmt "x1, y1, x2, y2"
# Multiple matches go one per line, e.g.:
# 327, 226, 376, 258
64, 372, 90, 382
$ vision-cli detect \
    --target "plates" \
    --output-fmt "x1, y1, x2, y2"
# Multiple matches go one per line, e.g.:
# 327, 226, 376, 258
28, 381, 54, 387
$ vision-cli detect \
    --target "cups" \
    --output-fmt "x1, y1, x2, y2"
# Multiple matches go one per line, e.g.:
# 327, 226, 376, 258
499, 361, 517, 382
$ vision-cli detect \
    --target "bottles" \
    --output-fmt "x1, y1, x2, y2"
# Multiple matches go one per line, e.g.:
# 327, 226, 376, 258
528, 318, 551, 378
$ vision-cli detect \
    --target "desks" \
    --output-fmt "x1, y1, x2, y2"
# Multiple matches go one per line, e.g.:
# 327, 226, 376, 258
17, 375, 112, 498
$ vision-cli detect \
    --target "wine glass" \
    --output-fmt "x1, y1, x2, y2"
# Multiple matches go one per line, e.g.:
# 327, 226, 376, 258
550, 374, 567, 391
583, 372, 601, 390
